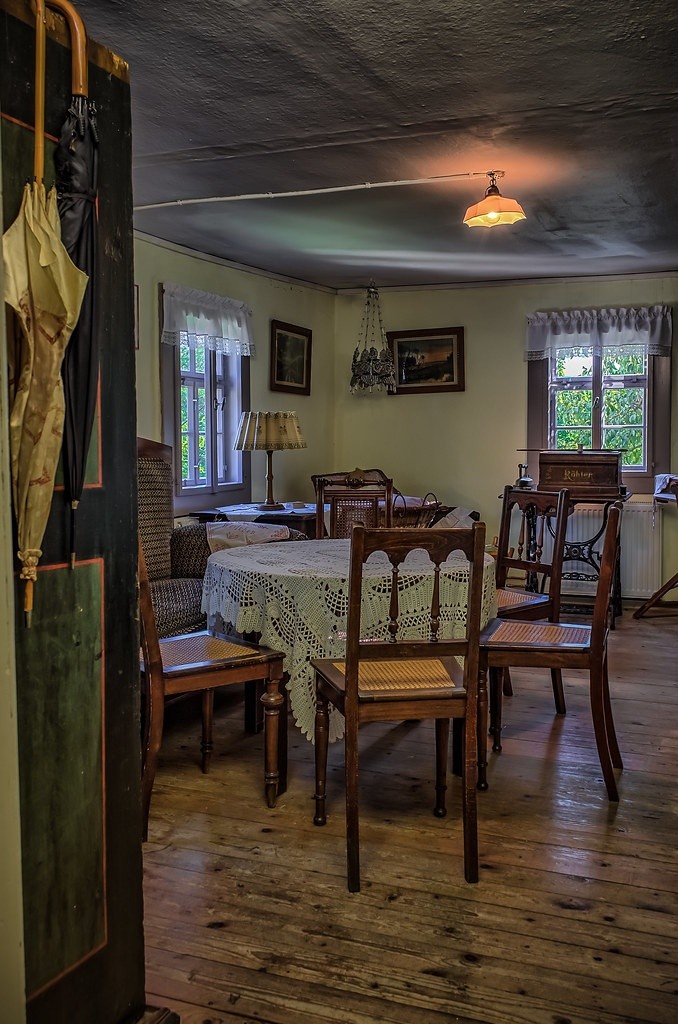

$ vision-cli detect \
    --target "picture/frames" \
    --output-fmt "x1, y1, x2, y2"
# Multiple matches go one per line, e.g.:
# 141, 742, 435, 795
271, 319, 312, 395
386, 326, 465, 395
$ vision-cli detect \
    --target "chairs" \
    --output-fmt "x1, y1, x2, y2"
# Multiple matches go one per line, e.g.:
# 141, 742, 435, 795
315, 478, 393, 539
495, 485, 571, 714
478, 500, 624, 803
136, 530, 286, 842
311, 468, 481, 529
311, 522, 487, 892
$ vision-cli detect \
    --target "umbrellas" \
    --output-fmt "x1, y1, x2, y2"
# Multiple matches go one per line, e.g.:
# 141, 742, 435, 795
2, 0, 100, 627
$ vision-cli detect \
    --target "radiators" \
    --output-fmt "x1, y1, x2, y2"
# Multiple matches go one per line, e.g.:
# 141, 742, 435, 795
532, 505, 664, 601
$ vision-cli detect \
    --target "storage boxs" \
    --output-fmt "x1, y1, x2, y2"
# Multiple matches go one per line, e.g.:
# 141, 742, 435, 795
536, 450, 624, 502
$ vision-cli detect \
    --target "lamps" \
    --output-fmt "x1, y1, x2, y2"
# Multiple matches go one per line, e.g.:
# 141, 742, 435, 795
231, 410, 308, 511
464, 172, 527, 227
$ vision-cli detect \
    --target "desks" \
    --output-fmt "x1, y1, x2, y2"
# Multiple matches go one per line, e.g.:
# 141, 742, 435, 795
200, 541, 498, 797
190, 502, 332, 540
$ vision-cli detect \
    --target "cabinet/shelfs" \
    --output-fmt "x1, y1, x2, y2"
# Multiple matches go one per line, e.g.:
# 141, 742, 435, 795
0, 0, 149, 1024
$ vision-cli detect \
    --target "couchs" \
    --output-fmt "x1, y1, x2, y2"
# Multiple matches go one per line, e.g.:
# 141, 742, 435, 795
136, 437, 311, 734
